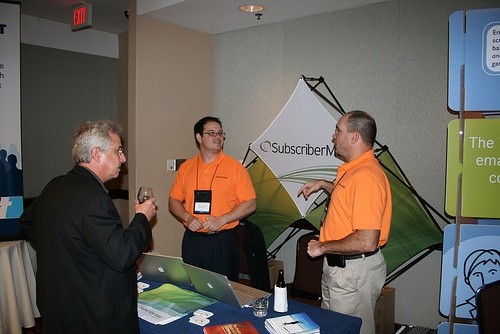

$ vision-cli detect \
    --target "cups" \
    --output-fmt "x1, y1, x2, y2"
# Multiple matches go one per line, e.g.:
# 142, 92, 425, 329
137, 187, 153, 204
251, 296, 269, 317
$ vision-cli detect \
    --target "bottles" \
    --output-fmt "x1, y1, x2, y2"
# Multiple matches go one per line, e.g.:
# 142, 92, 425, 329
274, 269, 288, 313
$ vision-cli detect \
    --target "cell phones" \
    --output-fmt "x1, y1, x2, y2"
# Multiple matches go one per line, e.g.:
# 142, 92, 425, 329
326, 254, 346, 268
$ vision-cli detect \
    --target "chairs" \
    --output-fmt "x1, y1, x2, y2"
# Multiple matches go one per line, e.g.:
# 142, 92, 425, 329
267, 231, 323, 309
474, 279, 500, 334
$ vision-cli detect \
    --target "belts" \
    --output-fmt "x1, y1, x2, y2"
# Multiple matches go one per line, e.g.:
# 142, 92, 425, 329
345, 248, 380, 260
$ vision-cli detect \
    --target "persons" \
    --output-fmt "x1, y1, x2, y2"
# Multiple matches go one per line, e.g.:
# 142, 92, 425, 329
17, 119, 156, 334
168, 117, 256, 282
297, 111, 392, 334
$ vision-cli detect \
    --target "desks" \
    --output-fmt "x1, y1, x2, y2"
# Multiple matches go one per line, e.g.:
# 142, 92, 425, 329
0, 239, 39, 334
268, 260, 284, 289
373, 286, 397, 334
137, 277, 362, 334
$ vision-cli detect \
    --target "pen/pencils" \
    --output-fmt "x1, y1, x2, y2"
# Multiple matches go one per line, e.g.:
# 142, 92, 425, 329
241, 305, 266, 308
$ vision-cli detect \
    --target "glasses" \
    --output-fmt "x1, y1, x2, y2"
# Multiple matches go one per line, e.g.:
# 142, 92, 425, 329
103, 148, 124, 155
202, 129, 225, 136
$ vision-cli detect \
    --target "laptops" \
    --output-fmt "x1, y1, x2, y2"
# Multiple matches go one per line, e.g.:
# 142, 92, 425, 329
181, 263, 272, 309
137, 254, 194, 286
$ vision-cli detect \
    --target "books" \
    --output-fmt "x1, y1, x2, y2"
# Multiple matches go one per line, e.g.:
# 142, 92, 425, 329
264, 313, 321, 334
202, 321, 260, 334
